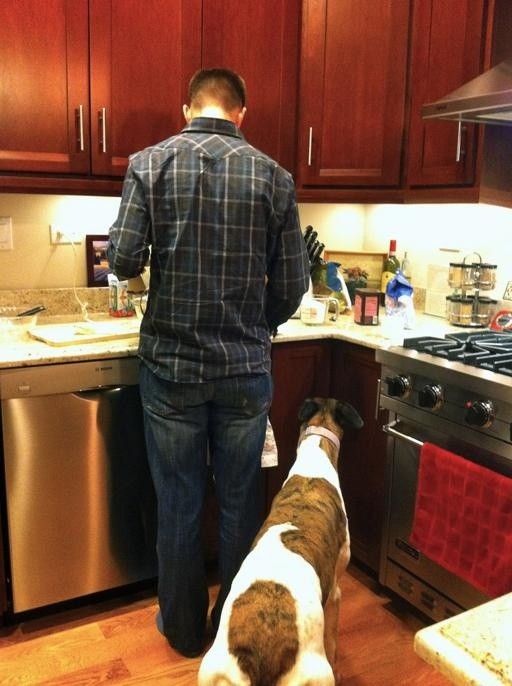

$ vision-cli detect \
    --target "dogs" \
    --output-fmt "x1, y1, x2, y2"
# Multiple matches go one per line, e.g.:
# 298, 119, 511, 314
196, 396, 365, 686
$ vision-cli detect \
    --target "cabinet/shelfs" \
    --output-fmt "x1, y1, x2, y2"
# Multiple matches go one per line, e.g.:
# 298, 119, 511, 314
402, 0, 497, 204
1, 1, 202, 196
295, 1, 409, 204
201, 1, 301, 182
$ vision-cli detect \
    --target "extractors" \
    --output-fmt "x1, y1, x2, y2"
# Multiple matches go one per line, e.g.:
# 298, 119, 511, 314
420, 54, 512, 128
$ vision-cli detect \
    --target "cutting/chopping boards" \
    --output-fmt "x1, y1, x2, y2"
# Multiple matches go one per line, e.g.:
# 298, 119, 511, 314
28, 317, 140, 346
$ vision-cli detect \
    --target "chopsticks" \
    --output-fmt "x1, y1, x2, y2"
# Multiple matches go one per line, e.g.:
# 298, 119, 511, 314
18, 304, 47, 318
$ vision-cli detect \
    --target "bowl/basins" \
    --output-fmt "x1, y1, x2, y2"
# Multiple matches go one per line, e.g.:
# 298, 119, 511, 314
0, 306, 38, 340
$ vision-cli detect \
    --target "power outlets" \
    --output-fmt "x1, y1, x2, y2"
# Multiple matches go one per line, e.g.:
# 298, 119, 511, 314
48, 223, 83, 244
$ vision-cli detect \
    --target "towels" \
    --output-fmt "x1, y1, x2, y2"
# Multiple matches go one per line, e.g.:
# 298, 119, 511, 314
408, 441, 512, 598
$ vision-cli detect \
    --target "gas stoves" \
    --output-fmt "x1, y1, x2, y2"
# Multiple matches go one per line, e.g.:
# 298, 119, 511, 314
377, 326, 512, 441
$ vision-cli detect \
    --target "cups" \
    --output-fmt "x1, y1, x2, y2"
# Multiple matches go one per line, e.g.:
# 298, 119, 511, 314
299, 293, 340, 324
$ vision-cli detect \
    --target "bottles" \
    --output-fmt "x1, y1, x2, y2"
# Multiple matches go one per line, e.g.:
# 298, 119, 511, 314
400, 250, 412, 284
445, 261, 499, 327
380, 238, 400, 309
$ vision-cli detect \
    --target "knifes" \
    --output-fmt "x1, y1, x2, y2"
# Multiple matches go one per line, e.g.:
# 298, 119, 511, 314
302, 222, 326, 263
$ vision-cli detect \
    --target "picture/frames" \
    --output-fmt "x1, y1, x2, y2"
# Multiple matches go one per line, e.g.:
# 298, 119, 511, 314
85, 234, 110, 287
323, 251, 389, 293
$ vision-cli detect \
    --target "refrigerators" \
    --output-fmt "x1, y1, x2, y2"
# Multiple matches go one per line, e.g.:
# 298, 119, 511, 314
1, 356, 160, 617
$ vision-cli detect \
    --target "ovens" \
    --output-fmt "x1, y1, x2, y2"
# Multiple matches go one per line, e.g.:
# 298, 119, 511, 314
378, 409, 511, 623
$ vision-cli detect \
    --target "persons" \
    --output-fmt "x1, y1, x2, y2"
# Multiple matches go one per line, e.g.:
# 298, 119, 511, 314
104, 67, 308, 659
